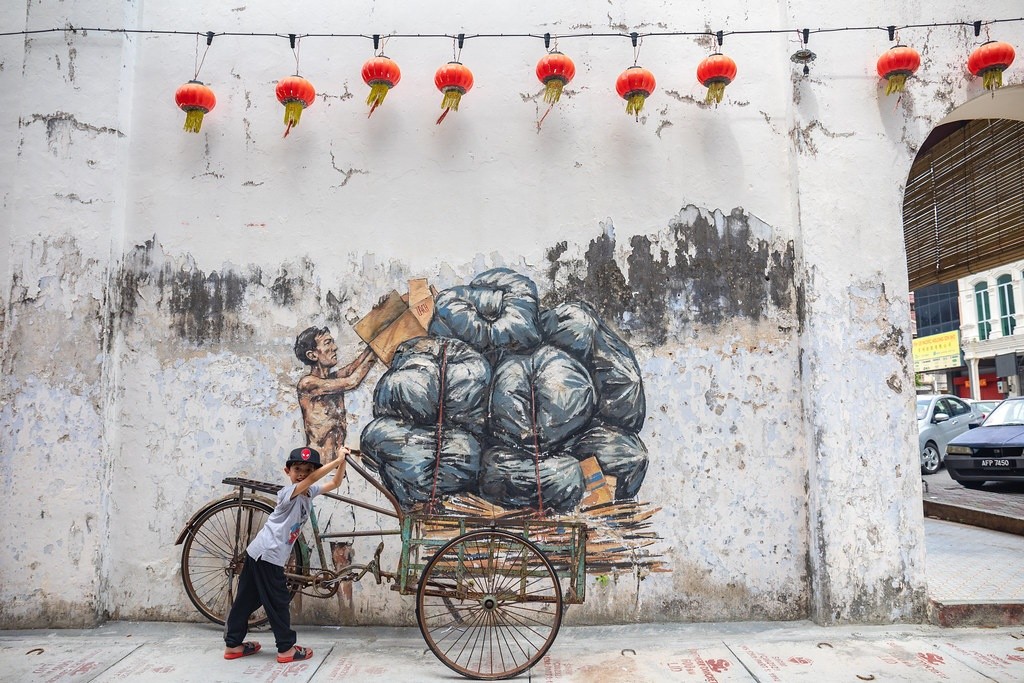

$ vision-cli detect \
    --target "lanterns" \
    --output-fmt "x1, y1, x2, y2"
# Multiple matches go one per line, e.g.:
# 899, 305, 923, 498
876, 44, 920, 97
433, 60, 475, 123
966, 39, 1016, 93
175, 79, 215, 134
694, 52, 736, 105
538, 51, 574, 106
617, 64, 657, 115
275, 75, 317, 137
362, 52, 400, 120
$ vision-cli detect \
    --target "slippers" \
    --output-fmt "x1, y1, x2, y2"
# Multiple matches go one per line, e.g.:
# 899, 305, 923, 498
277, 646, 313, 663
224, 641, 261, 659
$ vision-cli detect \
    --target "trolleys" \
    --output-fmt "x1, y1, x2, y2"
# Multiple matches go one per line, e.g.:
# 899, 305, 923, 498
175, 450, 588, 671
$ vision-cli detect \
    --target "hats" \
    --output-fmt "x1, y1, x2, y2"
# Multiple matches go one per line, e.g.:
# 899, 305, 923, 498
286, 447, 323, 469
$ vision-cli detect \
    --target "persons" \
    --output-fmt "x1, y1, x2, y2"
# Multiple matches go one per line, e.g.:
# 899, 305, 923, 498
222, 443, 354, 663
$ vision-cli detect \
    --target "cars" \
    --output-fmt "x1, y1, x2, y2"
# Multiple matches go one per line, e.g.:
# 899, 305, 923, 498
964, 398, 1006, 427
944, 396, 1024, 497
912, 394, 986, 475
